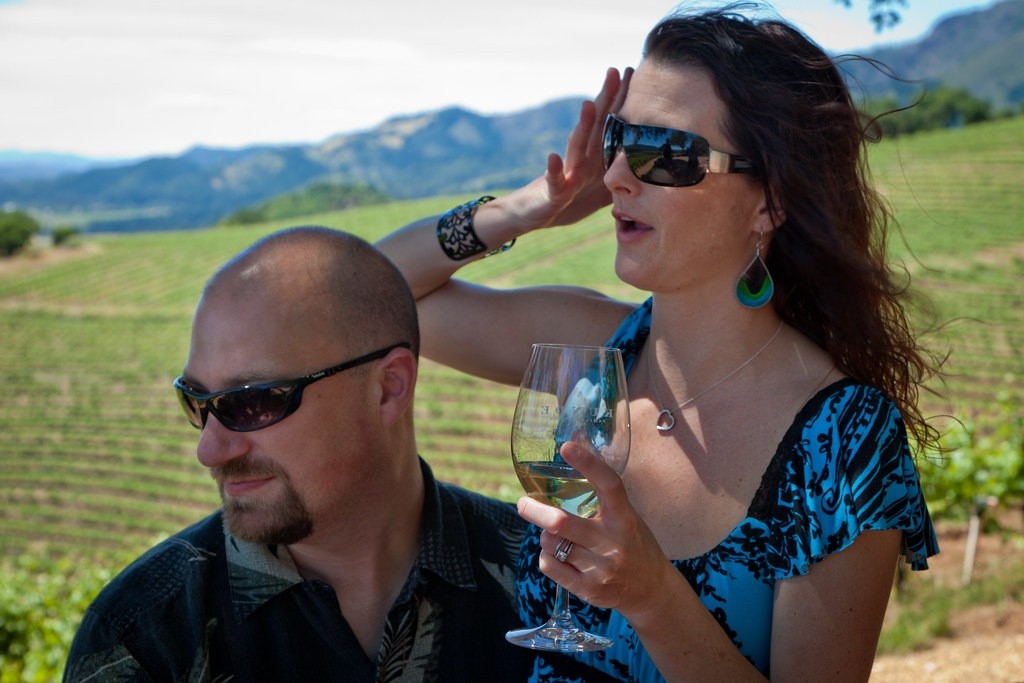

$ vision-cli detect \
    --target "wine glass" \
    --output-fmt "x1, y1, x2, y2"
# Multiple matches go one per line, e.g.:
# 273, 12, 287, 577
505, 344, 631, 653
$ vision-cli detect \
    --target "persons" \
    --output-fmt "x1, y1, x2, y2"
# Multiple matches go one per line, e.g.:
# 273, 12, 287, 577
371, 1, 992, 683
62, 225, 532, 683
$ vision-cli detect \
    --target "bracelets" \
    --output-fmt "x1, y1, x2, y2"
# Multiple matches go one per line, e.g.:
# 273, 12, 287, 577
435, 194, 517, 263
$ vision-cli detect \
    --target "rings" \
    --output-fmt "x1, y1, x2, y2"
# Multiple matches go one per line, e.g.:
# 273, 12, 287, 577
552, 538, 575, 563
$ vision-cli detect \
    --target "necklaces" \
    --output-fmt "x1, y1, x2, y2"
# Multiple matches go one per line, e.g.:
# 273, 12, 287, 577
647, 321, 784, 432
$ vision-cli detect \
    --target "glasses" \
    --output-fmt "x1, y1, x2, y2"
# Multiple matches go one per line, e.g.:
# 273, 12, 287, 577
171, 342, 413, 432
601, 112, 763, 187
